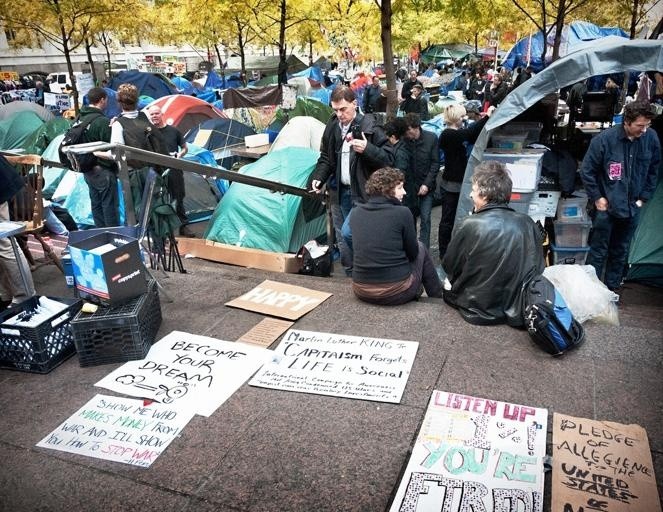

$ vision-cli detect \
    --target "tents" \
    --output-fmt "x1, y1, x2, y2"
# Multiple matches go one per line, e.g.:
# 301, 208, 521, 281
451, 39, 663, 235
0, 54, 312, 254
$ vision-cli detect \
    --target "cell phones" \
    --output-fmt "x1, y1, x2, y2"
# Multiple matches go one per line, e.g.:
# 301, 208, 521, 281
351, 124, 361, 140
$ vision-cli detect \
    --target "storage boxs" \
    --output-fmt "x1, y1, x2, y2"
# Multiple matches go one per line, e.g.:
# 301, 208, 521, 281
67, 230, 148, 306
481, 122, 593, 266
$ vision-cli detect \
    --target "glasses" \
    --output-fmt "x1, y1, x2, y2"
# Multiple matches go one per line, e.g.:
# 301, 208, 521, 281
629, 121, 652, 129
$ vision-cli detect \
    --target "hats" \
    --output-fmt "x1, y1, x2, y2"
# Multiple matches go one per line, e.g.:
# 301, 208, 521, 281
464, 100, 481, 113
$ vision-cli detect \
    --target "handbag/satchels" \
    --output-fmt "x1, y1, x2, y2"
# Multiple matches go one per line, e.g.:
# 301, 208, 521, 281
399, 99, 408, 110
299, 245, 332, 277
524, 276, 586, 358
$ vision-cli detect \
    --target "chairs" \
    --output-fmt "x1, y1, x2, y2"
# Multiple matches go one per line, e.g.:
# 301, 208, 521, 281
2, 153, 64, 277
68, 167, 174, 302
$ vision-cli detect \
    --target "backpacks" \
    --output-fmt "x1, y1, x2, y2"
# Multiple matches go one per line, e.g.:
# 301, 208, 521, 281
58, 111, 103, 173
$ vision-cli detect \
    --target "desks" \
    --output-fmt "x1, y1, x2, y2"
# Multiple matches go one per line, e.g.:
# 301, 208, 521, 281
0, 222, 32, 296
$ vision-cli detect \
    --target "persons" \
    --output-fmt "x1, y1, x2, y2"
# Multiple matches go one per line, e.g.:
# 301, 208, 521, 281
0, 151, 36, 309
441, 160, 546, 327
579, 99, 661, 292
566, 70, 663, 129
341, 167, 443, 306
312, 50, 533, 278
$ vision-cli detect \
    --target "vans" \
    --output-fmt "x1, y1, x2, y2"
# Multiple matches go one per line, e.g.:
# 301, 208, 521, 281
45, 72, 81, 91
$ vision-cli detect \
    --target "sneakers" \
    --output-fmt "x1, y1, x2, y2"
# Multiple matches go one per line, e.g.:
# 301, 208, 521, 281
610, 289, 625, 308
180, 226, 195, 237
